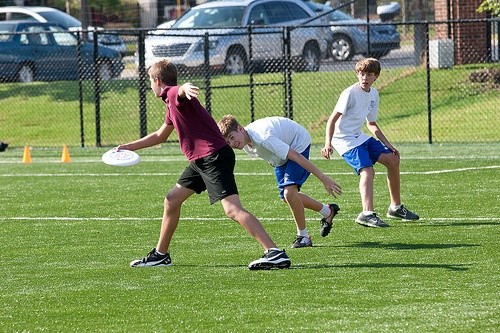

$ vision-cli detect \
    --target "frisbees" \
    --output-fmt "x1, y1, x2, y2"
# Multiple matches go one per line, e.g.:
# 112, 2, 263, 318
101, 148, 141, 167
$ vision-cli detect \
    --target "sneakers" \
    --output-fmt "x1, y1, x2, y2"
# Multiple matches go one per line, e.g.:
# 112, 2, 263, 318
291, 234, 313, 248
356, 211, 390, 228
320, 203, 341, 238
130, 248, 172, 267
248, 248, 292, 270
387, 204, 420, 220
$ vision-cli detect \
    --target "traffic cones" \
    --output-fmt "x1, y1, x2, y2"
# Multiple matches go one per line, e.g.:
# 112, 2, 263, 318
22, 146, 32, 163
62, 144, 70, 162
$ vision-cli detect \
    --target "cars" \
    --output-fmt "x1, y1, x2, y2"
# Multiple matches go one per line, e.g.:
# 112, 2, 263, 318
0, 20, 125, 83
313, 3, 401, 61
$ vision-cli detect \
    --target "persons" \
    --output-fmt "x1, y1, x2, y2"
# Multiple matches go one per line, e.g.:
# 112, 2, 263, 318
114, 60, 291, 270
321, 57, 419, 229
217, 115, 343, 248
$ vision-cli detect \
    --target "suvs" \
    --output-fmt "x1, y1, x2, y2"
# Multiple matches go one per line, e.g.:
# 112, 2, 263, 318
135, 0, 328, 77
0, 6, 128, 60
